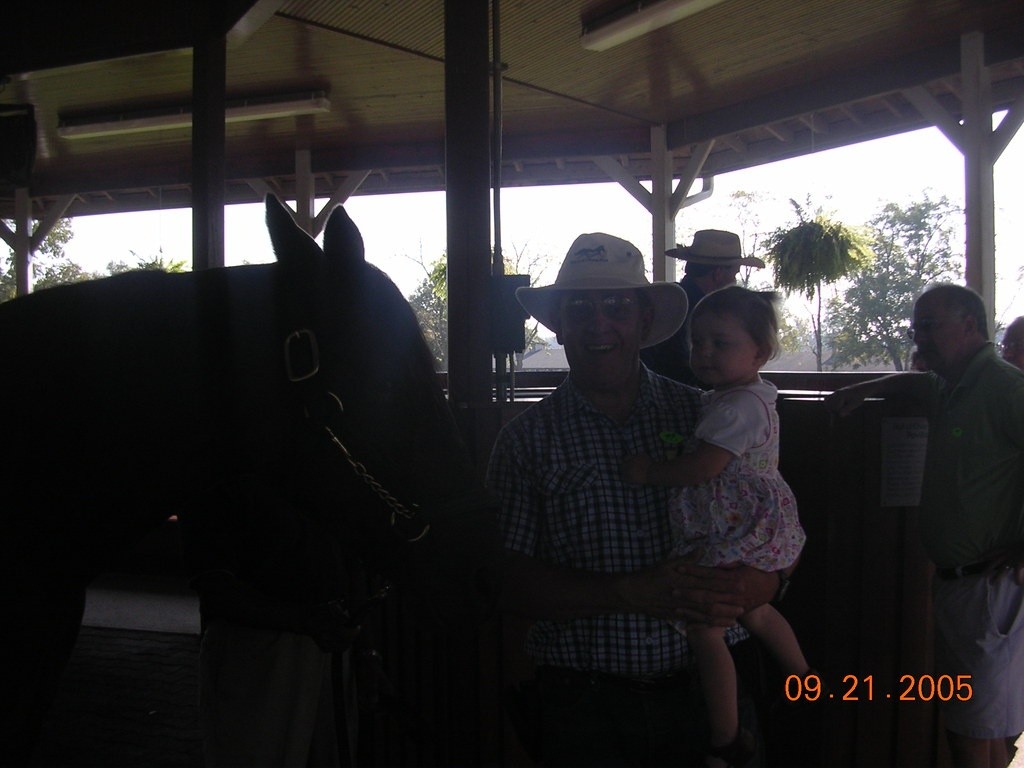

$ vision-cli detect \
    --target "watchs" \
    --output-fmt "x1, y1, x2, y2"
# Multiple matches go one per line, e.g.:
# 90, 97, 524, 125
771, 569, 790, 607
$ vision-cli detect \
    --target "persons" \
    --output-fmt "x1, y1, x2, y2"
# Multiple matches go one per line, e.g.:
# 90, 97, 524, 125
620, 286, 830, 767
484, 232, 1024, 768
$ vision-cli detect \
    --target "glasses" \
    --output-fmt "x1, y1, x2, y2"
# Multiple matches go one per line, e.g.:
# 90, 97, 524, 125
556, 293, 648, 322
905, 317, 942, 339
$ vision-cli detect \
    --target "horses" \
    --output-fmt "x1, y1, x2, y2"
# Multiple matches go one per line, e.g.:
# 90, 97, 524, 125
0, 193, 494, 768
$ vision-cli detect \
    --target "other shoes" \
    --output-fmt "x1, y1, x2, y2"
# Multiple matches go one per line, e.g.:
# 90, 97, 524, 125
692, 723, 757, 768
778, 668, 828, 715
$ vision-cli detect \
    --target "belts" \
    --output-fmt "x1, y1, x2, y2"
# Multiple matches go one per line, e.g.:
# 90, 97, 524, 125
938, 553, 1009, 582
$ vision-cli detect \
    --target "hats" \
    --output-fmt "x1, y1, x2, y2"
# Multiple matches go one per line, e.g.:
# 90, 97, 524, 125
663, 228, 766, 271
513, 232, 689, 352
